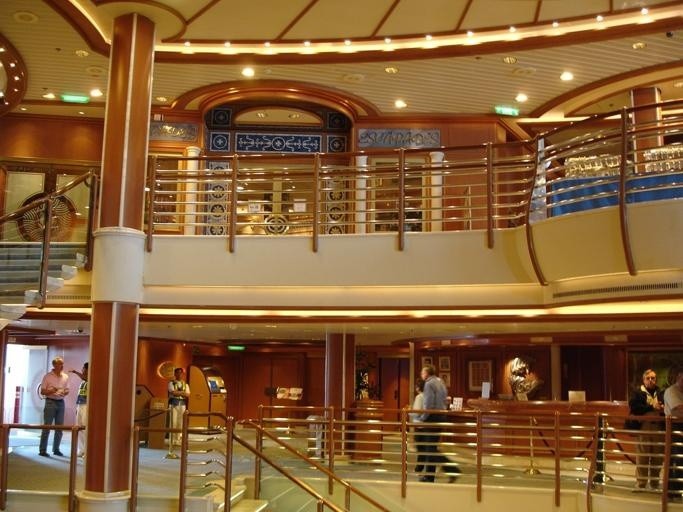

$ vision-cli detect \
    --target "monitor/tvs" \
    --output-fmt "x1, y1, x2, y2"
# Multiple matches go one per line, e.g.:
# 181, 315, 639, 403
497, 393, 513, 400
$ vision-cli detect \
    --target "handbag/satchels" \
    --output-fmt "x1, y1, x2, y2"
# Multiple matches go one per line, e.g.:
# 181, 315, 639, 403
624, 420, 641, 430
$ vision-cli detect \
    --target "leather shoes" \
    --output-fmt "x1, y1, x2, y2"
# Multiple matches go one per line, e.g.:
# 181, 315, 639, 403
419, 476, 434, 482
448, 472, 462, 483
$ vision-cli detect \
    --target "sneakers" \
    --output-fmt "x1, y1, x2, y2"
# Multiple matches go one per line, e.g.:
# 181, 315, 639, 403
40, 451, 49, 457
54, 450, 63, 456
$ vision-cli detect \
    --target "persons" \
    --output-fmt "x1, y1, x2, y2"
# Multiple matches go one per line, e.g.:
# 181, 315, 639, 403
419, 364, 462, 483
69, 361, 88, 458
628, 369, 665, 489
168, 368, 191, 443
660, 369, 683, 501
39, 357, 70, 456
407, 378, 426, 473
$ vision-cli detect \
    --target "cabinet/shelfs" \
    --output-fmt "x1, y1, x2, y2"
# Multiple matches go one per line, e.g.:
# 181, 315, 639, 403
353, 399, 385, 464
